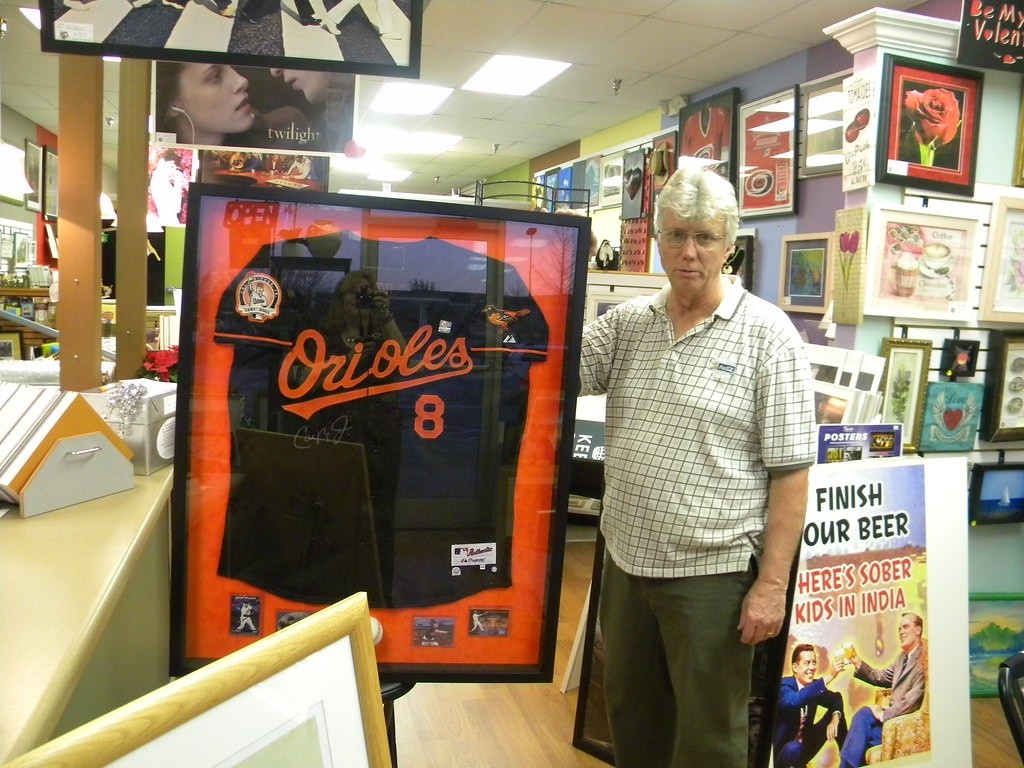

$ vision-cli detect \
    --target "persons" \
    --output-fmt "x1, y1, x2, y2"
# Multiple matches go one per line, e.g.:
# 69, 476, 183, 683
211, 153, 319, 180
580, 164, 817, 768
421, 620, 452, 646
838, 612, 926, 768
236, 600, 257, 633
156, 61, 354, 152
233, 270, 411, 605
280, 616, 294, 629
774, 643, 848, 768
469, 610, 490, 632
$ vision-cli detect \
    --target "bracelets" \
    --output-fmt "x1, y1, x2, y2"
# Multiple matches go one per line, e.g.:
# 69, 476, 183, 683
381, 314, 393, 324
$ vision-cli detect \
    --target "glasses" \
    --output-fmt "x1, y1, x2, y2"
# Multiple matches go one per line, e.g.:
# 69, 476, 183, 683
657, 228, 725, 251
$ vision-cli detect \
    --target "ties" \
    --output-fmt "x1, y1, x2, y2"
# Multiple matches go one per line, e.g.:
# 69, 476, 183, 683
898, 653, 908, 678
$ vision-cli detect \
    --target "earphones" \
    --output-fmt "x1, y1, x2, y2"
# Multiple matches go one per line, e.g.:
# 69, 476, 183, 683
171, 106, 186, 114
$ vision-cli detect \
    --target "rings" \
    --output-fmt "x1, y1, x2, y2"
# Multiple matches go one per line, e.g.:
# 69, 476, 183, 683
767, 632, 772, 635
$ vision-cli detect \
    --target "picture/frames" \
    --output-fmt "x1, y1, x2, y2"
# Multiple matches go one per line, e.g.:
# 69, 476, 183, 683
545, 72, 843, 315
171, 180, 592, 682
836, 55, 1024, 526
0, 593, 395, 768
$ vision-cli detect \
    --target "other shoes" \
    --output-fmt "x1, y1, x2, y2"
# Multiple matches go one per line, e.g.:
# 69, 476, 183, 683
220, 5, 236, 17
63, 0, 89, 11
162, 0, 184, 10
240, 9, 260, 25
132, 1, 152, 9
302, 15, 322, 25
323, 12, 341, 35
382, 33, 401, 40
